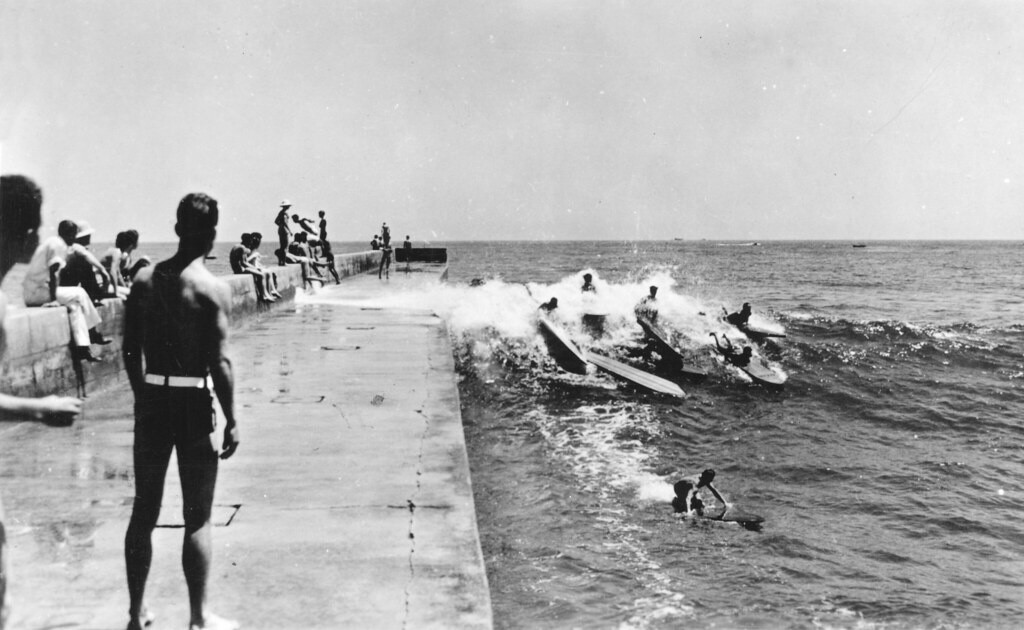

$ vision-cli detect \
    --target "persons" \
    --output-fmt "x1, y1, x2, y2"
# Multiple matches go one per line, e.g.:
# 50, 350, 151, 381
59, 220, 117, 300
247, 232, 282, 299
403, 234, 412, 273
318, 240, 340, 284
122, 192, 240, 630
370, 235, 380, 250
94, 232, 133, 301
291, 214, 318, 236
122, 229, 151, 285
0, 172, 84, 630
230, 233, 276, 302
582, 274, 595, 292
21, 220, 113, 361
709, 332, 753, 366
290, 232, 333, 277
724, 301, 752, 324
537, 296, 560, 311
378, 237, 383, 247
379, 244, 393, 279
275, 250, 332, 287
640, 285, 658, 304
319, 210, 329, 257
673, 468, 727, 514
274, 199, 293, 266
380, 222, 390, 246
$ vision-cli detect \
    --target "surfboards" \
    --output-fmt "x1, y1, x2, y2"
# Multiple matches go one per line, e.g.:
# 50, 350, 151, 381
587, 352, 687, 400
539, 317, 588, 366
676, 329, 789, 386
694, 504, 765, 524
717, 317, 788, 338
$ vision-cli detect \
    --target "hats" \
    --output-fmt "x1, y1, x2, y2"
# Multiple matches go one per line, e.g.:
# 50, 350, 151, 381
280, 200, 291, 207
76, 226, 93, 247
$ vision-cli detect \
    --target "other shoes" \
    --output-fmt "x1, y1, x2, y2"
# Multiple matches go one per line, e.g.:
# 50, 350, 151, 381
76, 347, 103, 362
90, 333, 113, 345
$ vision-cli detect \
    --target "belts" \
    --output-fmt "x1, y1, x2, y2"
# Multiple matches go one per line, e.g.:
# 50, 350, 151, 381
144, 374, 215, 389
